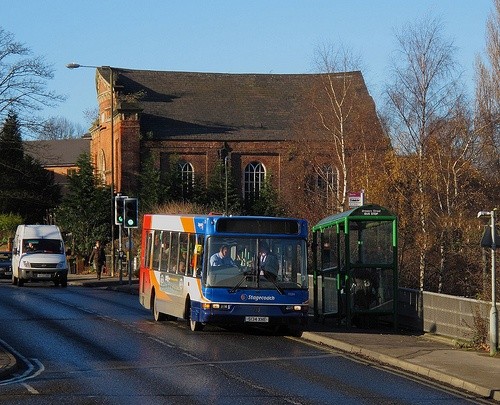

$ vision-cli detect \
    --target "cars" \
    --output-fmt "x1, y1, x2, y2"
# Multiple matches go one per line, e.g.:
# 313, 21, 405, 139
0, 251, 12, 279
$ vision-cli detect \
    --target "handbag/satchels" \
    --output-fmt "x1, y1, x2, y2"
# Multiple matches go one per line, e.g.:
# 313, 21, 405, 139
103, 267, 105, 273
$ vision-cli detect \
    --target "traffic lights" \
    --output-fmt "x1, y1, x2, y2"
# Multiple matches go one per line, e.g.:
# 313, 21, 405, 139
124, 198, 139, 228
115, 196, 128, 224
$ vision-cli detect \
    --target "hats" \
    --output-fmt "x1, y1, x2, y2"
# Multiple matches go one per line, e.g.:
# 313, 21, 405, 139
180, 248, 187, 252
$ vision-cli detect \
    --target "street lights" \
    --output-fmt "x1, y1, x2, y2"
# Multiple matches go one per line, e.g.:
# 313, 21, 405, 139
67, 63, 116, 279
477, 210, 498, 357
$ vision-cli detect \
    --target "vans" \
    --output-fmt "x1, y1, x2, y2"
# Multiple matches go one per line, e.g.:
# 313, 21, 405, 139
11, 224, 71, 287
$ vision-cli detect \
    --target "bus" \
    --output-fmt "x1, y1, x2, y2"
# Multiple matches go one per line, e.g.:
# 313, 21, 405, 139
139, 212, 310, 335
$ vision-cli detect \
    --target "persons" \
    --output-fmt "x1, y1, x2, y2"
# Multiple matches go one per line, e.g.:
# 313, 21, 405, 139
89, 241, 108, 279
26, 243, 34, 252
152, 234, 279, 281
